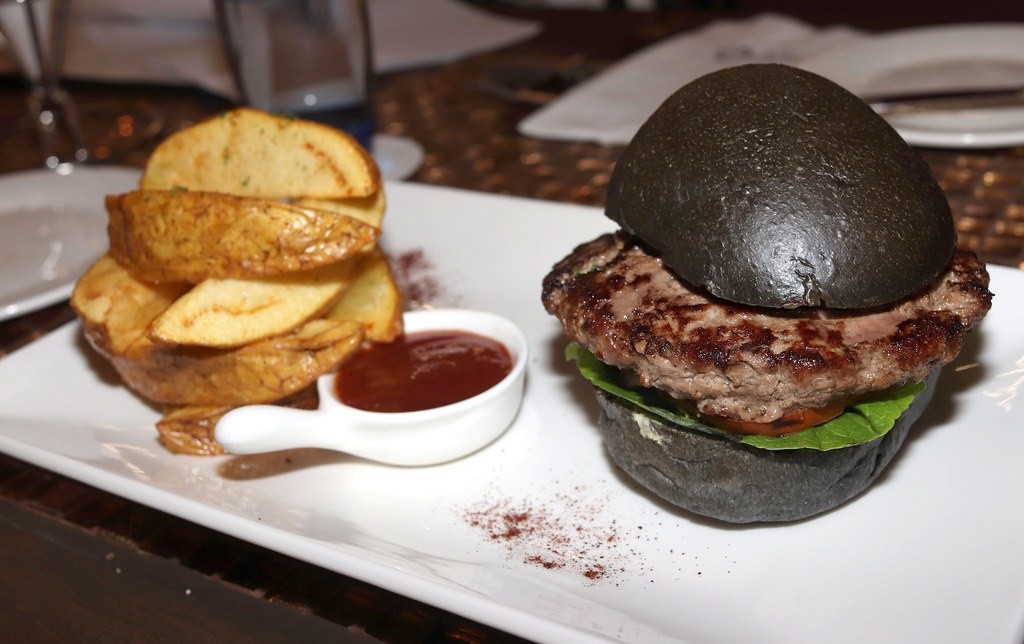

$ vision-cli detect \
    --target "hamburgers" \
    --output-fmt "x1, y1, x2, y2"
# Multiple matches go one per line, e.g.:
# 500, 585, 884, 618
541, 63, 993, 524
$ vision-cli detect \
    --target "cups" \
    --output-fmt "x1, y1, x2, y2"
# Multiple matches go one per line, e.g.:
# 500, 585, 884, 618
216, 0, 375, 155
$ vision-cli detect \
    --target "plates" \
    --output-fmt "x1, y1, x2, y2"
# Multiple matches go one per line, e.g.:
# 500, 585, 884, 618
0, 167, 141, 318
0, 179, 1024, 644
364, 136, 425, 178
799, 23, 1024, 148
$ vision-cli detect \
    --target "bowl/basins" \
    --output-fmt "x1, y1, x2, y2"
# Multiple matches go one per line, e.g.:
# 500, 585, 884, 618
214, 308, 529, 467
